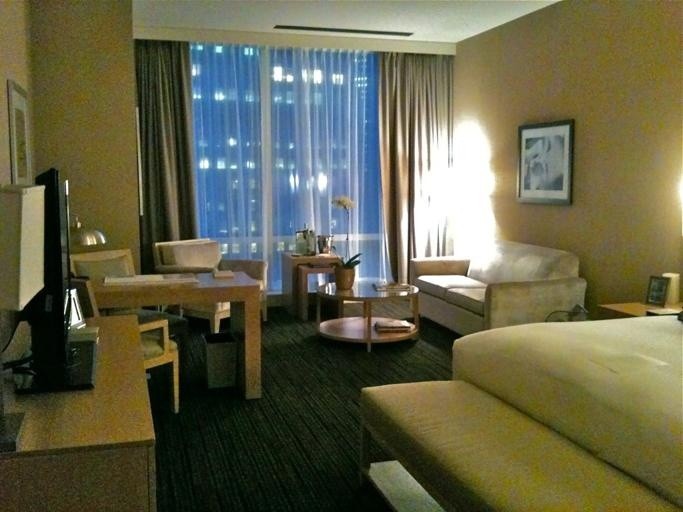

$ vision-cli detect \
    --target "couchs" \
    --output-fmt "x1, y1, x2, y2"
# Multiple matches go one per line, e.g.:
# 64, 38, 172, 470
407, 237, 589, 334
151, 238, 270, 334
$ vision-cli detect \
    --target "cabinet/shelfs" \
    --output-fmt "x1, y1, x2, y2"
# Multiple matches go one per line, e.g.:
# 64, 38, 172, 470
0, 313, 157, 512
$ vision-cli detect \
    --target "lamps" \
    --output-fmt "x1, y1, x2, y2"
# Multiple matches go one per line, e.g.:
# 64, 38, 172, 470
77, 230, 108, 246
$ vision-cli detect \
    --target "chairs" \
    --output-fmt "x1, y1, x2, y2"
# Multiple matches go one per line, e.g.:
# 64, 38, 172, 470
67, 276, 182, 415
68, 248, 186, 340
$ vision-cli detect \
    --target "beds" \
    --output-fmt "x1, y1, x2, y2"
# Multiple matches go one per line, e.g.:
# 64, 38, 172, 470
359, 316, 683, 512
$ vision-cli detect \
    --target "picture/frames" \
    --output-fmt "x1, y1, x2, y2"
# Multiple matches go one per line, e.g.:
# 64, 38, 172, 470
514, 118, 576, 205
644, 272, 671, 308
8, 81, 31, 185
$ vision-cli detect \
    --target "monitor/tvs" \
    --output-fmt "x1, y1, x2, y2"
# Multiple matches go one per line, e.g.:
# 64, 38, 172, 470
16, 168, 93, 393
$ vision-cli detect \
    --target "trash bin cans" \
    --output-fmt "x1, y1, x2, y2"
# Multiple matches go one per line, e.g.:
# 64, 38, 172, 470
201, 331, 239, 390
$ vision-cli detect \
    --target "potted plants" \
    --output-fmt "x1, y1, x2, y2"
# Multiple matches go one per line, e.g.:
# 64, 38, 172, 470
329, 250, 364, 290
